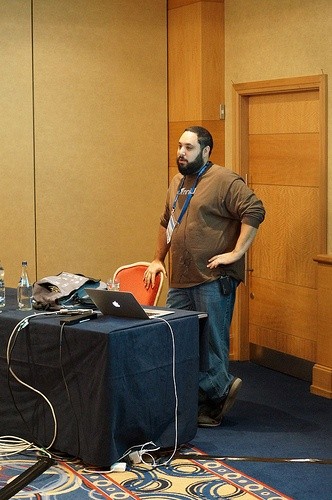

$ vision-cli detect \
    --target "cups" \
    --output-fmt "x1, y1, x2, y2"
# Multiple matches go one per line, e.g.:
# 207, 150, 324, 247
106, 277, 120, 291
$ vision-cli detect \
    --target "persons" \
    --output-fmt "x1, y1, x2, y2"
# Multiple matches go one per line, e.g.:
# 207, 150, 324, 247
142, 125, 266, 428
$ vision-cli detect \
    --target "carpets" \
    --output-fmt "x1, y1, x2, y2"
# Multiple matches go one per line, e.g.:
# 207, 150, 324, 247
0, 443, 291, 500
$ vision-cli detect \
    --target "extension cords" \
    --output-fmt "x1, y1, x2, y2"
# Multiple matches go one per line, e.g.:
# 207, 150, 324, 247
123, 451, 160, 465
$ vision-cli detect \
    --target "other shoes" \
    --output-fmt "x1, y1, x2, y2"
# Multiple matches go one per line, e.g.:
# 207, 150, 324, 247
197, 377, 242, 427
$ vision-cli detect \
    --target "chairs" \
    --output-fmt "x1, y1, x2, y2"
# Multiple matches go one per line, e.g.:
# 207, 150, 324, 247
113, 261, 163, 307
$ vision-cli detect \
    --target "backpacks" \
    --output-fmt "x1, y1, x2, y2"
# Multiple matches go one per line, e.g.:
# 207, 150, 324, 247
32, 271, 108, 311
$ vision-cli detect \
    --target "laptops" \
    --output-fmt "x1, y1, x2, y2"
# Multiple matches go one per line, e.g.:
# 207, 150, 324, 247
84, 288, 175, 320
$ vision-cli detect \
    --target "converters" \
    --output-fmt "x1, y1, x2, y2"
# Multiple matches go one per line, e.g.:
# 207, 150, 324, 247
60, 312, 97, 326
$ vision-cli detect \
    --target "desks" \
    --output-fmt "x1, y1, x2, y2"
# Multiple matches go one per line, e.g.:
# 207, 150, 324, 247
0, 290, 209, 471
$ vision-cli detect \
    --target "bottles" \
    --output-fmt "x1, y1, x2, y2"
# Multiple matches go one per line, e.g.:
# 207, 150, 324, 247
0, 263, 6, 306
17, 262, 33, 311
